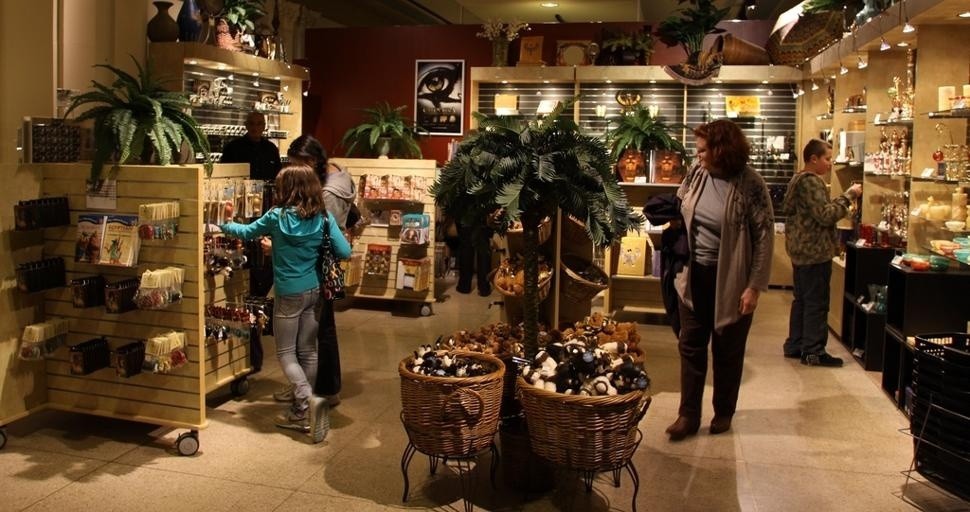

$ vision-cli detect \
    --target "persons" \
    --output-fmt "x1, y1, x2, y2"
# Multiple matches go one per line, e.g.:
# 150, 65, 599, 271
258, 134, 358, 409
215, 109, 284, 339
214, 162, 354, 447
454, 128, 494, 299
660, 116, 777, 440
780, 137, 863, 368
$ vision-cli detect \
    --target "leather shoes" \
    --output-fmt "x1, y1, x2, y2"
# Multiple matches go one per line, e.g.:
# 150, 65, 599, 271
667, 415, 700, 435
710, 415, 732, 432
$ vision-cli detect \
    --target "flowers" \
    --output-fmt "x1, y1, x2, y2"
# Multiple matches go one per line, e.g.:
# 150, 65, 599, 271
474, 14, 532, 39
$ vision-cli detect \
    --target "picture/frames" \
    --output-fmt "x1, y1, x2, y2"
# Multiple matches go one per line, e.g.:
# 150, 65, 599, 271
412, 58, 466, 137
518, 35, 544, 64
554, 39, 593, 66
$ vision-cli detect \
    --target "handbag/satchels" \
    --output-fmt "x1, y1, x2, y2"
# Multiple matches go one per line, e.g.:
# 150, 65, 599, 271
321, 250, 345, 300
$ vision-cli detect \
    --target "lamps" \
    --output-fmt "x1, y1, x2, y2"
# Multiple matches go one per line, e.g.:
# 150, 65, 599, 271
226, 51, 312, 97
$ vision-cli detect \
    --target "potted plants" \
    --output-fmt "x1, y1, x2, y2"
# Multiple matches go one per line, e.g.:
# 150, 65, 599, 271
332, 101, 431, 159
213, 0, 268, 54
425, 88, 651, 496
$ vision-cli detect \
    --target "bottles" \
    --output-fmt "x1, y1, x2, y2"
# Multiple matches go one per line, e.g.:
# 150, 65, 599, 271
942, 144, 969, 182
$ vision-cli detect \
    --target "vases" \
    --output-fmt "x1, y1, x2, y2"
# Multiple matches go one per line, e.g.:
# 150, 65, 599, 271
488, 37, 512, 66
147, 0, 182, 41
709, 32, 770, 65
176, 0, 211, 45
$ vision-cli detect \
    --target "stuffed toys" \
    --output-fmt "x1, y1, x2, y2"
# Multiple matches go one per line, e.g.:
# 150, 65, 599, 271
408, 252, 653, 395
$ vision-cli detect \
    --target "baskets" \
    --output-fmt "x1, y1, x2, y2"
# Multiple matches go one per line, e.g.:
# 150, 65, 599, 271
566, 211, 595, 259
506, 215, 552, 249
495, 262, 554, 305
560, 254, 608, 305
398, 336, 520, 455
517, 371, 647, 469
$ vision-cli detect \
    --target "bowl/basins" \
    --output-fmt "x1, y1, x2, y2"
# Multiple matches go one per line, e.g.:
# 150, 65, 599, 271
898, 221, 969, 274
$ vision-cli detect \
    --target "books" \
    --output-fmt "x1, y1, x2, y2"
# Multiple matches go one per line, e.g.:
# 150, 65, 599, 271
618, 207, 670, 276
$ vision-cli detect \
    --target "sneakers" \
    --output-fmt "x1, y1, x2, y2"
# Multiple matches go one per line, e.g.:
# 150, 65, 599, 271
274, 384, 341, 443
802, 350, 844, 367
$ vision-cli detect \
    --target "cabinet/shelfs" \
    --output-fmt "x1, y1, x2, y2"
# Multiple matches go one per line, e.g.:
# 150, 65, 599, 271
882, 260, 970, 424
906, 0, 969, 263
825, 254, 847, 338
840, 239, 896, 372
602, 183, 685, 325
470, 65, 799, 228
790, 0, 916, 247
146, 42, 313, 169
